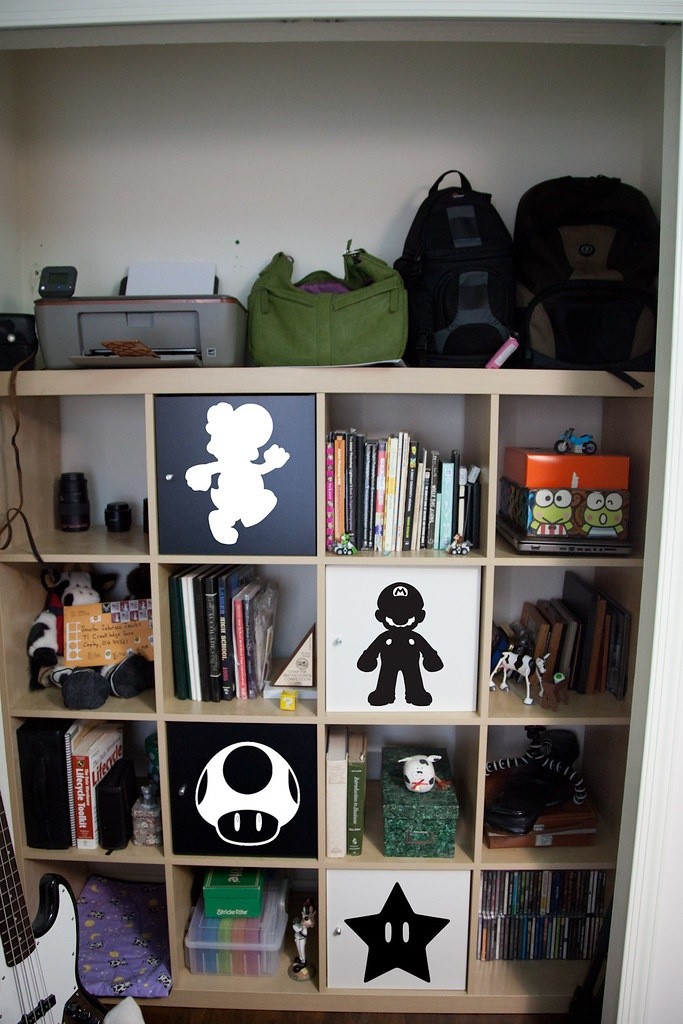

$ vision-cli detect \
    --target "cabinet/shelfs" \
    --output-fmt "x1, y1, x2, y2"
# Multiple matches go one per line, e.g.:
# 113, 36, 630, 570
1, 369, 655, 1014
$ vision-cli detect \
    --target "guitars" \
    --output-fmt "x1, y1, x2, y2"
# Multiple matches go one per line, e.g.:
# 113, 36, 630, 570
0, 793, 146, 1024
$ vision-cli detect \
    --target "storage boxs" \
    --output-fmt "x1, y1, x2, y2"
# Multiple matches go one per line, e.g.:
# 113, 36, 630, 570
185, 866, 289, 976
485, 769, 598, 848
381, 747, 459, 858
500, 447, 630, 539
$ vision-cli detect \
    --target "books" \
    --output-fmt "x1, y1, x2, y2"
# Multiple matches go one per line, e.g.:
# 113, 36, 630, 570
325, 427, 480, 551
326, 724, 368, 858
477, 870, 616, 962
490, 570, 632, 701
16, 719, 138, 850
168, 564, 279, 703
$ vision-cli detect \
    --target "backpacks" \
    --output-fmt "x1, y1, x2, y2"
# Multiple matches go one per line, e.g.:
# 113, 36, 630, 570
514, 175, 661, 389
393, 169, 533, 369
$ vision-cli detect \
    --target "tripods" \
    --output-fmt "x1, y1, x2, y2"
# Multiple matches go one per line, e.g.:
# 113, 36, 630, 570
485, 725, 586, 811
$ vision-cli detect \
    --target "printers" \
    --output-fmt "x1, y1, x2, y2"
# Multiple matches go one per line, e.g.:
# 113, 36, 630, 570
30, 271, 250, 369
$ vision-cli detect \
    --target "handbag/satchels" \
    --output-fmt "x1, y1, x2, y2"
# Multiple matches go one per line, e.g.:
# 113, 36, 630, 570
246, 239, 409, 367
